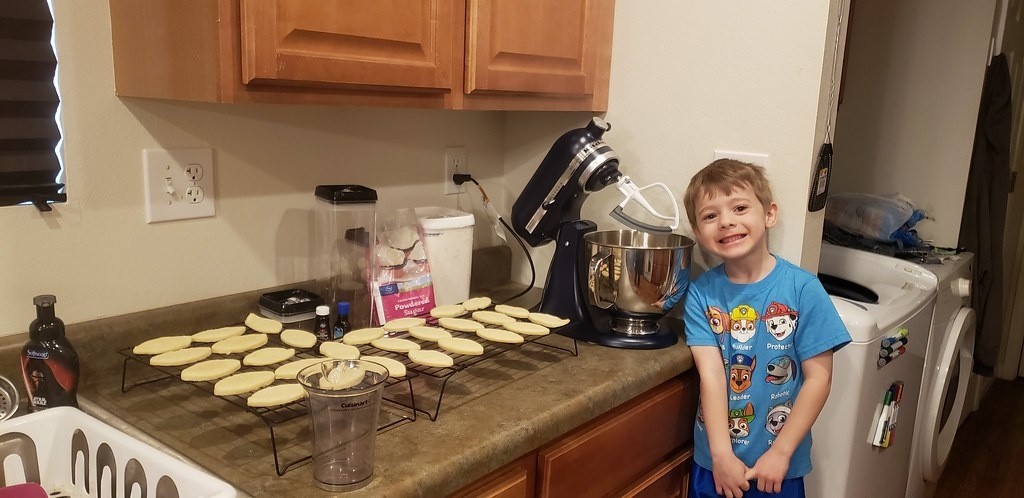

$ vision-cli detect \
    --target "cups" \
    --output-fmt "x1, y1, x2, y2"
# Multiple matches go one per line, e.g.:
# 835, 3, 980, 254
297, 359, 389, 492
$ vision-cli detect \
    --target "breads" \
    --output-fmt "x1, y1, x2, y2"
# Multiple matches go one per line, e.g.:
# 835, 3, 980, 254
133, 297, 570, 407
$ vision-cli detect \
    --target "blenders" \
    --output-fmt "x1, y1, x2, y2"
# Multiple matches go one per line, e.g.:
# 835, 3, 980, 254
511, 117, 696, 349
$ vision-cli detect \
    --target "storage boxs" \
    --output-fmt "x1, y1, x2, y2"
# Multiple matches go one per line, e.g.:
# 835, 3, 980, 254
396, 205, 474, 307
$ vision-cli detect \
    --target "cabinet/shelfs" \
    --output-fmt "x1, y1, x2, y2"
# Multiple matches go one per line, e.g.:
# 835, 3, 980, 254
447, 373, 700, 498
109, 0, 615, 112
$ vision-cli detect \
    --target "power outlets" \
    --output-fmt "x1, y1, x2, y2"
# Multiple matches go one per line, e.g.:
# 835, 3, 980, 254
443, 145, 469, 196
142, 145, 217, 225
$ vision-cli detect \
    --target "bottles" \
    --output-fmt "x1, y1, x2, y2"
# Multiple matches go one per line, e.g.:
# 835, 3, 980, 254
314, 306, 331, 356
333, 302, 350, 339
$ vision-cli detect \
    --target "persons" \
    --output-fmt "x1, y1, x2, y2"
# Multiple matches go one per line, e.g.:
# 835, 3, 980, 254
652, 249, 689, 309
633, 247, 646, 290
681, 158, 852, 498
